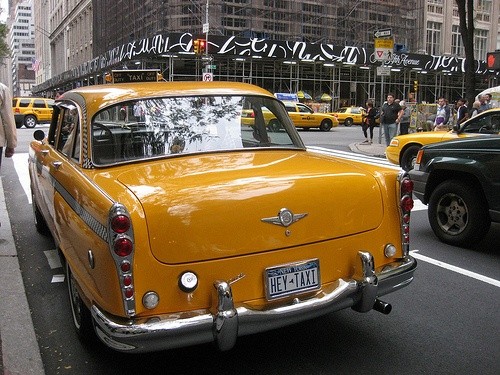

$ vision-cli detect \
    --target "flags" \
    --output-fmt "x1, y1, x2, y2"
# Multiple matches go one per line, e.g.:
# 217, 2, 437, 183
31, 58, 40, 72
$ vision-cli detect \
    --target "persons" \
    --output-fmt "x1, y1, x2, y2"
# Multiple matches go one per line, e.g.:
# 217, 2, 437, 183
456, 99, 469, 130
0, 83, 17, 227
362, 100, 374, 143
380, 94, 404, 155
434, 97, 450, 130
384, 99, 410, 134
469, 94, 492, 119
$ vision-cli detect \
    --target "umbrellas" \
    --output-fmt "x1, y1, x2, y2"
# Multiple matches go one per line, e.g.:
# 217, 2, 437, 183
297, 90, 312, 99
315, 93, 332, 101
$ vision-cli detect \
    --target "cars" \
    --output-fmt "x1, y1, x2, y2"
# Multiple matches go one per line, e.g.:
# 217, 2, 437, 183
27, 81, 417, 355
385, 107, 500, 246
241, 102, 380, 132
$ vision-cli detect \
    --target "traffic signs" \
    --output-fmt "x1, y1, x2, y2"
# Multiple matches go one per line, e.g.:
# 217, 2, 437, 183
202, 55, 214, 61
373, 28, 392, 39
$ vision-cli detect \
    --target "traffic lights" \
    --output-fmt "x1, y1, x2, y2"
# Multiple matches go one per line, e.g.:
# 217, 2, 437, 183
193, 38, 206, 54
486, 51, 500, 70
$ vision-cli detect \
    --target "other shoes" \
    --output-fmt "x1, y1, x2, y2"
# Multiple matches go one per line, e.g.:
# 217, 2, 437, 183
362, 139, 367, 143
369, 140, 372, 143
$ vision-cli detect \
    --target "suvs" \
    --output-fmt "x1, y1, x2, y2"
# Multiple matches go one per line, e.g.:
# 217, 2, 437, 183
12, 97, 56, 128
